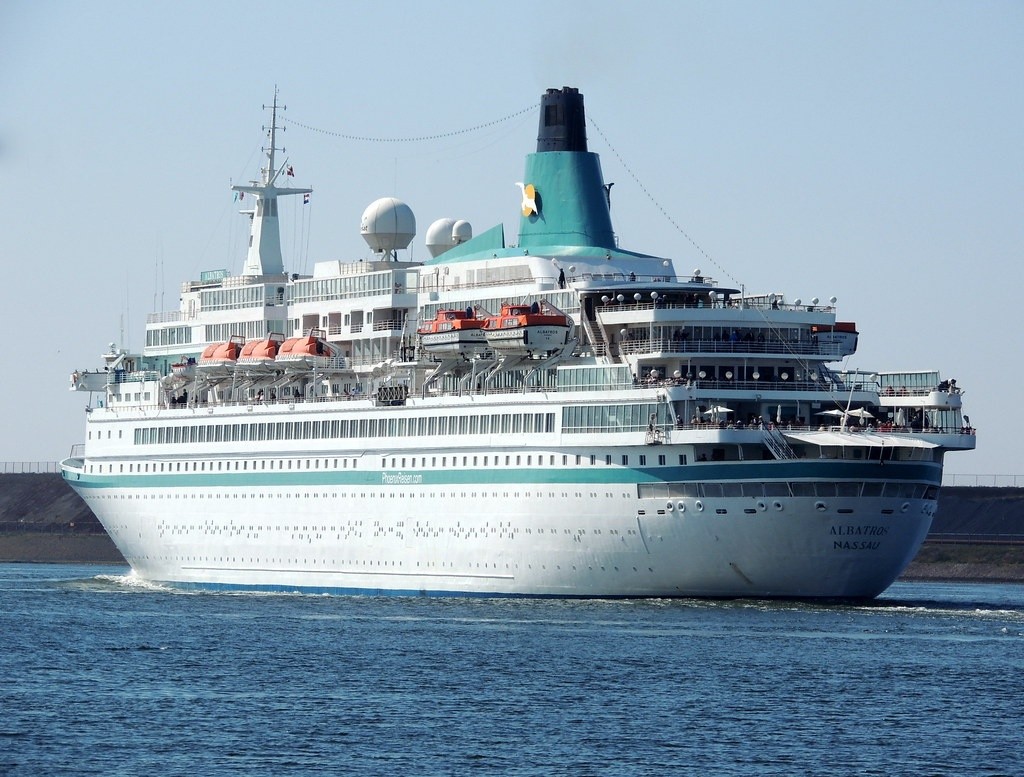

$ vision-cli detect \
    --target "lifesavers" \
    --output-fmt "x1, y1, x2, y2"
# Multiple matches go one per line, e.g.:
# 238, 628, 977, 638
395, 283, 401, 293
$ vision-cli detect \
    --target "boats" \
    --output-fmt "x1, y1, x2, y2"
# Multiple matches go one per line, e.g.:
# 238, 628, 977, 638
197, 335, 248, 375
416, 307, 489, 357
236, 332, 286, 369
168, 355, 199, 378
272, 326, 341, 369
482, 303, 569, 354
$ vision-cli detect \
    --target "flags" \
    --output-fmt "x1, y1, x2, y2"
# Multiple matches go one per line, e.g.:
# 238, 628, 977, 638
239, 191, 244, 201
287, 165, 294, 177
304, 194, 309, 205
233, 192, 238, 204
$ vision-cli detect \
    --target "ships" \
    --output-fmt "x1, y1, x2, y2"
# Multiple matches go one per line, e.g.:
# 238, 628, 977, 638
58, 77, 978, 603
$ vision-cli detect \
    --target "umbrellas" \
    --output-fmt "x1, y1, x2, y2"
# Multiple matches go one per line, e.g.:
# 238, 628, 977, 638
704, 404, 874, 418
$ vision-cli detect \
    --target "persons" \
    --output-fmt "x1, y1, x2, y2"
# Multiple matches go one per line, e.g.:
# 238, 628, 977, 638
257, 389, 263, 401
352, 388, 355, 396
710, 377, 717, 381
628, 271, 636, 281
311, 389, 314, 396
531, 301, 539, 314
271, 390, 276, 399
656, 295, 670, 309
726, 375, 910, 396
649, 412, 975, 434
294, 389, 300, 397
688, 281, 691, 283
644, 373, 674, 388
673, 328, 689, 341
192, 395, 198, 403
344, 389, 349, 395
558, 267, 565, 289
171, 389, 187, 404
675, 291, 711, 308
632, 373, 637, 385
772, 300, 779, 310
465, 305, 472, 319
714, 330, 766, 342
654, 278, 656, 282
